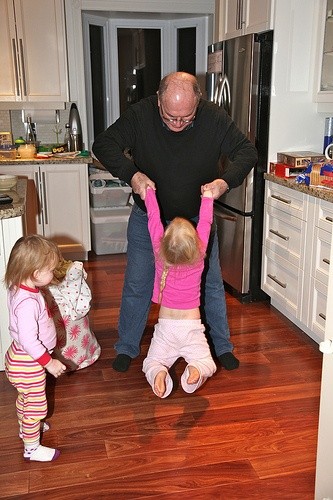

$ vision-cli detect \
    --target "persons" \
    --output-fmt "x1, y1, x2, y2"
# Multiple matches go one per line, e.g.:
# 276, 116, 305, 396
142, 186, 217, 398
4, 234, 67, 461
93, 72, 258, 372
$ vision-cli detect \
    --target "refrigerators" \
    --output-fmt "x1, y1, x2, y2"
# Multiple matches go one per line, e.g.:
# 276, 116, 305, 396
204, 32, 271, 304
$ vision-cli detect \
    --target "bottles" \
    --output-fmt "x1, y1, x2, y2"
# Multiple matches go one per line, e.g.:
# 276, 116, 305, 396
17, 145, 36, 159
64, 123, 71, 152
26, 116, 35, 142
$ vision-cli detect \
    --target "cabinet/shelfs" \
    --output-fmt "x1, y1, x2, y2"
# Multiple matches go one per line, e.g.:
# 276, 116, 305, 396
219, 0, 274, 42
260, 180, 333, 345
0, 164, 92, 371
0, 0, 69, 102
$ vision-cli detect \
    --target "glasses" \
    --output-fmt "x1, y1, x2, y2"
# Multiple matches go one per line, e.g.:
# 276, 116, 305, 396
159, 101, 197, 123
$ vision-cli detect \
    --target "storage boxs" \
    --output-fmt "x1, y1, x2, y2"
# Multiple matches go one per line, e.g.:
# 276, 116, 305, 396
269, 151, 325, 178
89, 167, 134, 255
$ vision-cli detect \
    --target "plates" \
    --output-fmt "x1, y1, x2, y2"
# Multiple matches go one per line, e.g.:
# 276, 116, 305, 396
36, 151, 80, 158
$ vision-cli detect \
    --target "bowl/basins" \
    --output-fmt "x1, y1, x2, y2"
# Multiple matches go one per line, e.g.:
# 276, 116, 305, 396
25, 141, 41, 153
0, 148, 16, 160
0, 174, 18, 191
